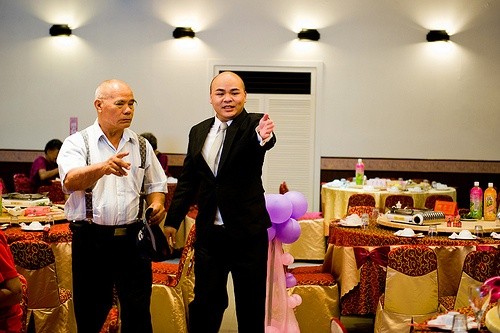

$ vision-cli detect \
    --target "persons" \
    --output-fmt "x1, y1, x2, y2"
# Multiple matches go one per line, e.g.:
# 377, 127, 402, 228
29, 138, 63, 193
0, 229, 23, 333
164, 72, 276, 333
57, 79, 168, 333
140, 133, 168, 174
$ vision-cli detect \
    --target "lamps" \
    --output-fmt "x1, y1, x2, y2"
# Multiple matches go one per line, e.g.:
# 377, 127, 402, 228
427, 30, 450, 42
298, 29, 320, 41
173, 27, 195, 39
49, 25, 71, 36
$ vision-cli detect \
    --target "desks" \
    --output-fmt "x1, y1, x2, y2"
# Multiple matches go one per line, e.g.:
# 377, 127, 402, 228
321, 180, 456, 236
322, 214, 500, 314
0, 204, 73, 294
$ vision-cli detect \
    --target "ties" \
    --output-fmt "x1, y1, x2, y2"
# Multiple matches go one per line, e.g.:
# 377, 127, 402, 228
207, 122, 228, 174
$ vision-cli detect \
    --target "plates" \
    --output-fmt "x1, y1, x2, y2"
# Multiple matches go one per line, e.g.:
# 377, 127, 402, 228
428, 320, 478, 329
21, 226, 45, 230
12, 193, 44, 199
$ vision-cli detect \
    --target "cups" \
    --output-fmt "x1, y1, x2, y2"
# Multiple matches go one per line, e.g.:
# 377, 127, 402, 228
474, 225, 483, 239
452, 314, 468, 333
427, 224, 438, 239
432, 181, 437, 190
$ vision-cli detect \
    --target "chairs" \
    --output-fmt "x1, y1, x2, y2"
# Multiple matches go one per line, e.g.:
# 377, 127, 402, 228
0, 173, 500, 333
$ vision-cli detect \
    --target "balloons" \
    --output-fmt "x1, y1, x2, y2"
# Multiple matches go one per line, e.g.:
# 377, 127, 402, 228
264, 191, 308, 308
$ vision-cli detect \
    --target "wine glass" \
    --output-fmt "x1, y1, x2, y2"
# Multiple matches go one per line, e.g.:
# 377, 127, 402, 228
467, 286, 491, 330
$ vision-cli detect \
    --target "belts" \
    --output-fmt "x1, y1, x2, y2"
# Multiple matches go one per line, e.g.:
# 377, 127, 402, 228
92, 223, 142, 237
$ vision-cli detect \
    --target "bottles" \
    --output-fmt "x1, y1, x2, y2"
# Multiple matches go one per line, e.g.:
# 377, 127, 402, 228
484, 183, 497, 222
470, 181, 482, 219
355, 159, 364, 189
